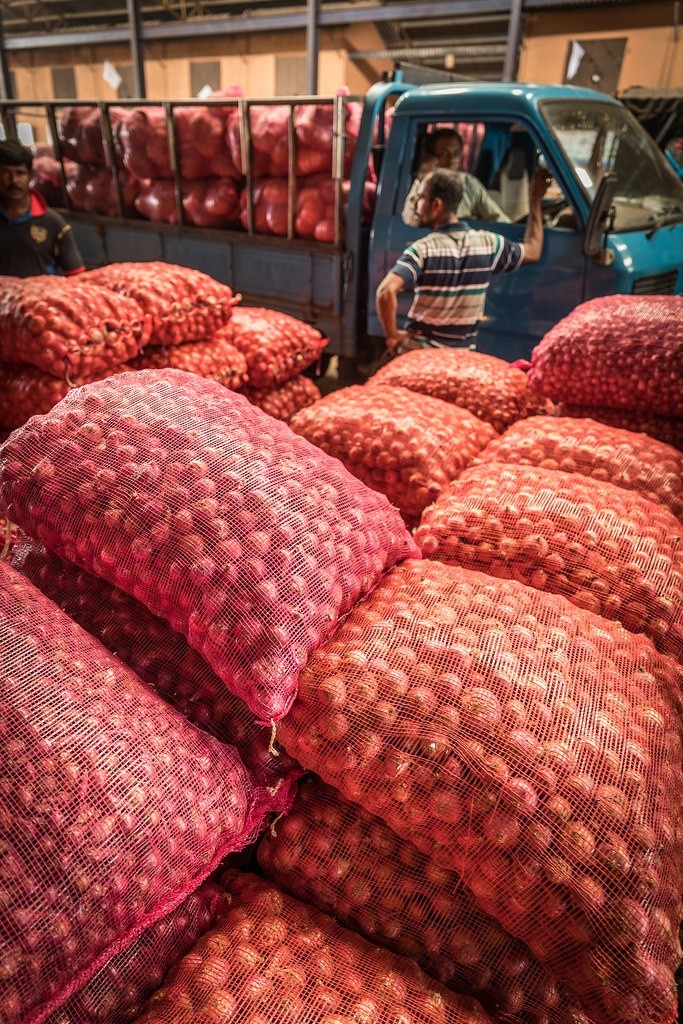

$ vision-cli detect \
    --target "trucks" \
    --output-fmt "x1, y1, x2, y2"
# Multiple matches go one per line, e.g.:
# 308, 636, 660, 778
0, 61, 683, 383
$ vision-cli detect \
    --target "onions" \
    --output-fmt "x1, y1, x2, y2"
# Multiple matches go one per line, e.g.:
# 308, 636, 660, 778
0, 259, 683, 1024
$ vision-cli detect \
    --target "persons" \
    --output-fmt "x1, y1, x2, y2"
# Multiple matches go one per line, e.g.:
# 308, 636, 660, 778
664, 132, 683, 182
376, 166, 549, 376
0, 140, 86, 279
401, 128, 512, 227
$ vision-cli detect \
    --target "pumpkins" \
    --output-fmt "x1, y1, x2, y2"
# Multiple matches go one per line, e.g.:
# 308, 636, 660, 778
27, 101, 482, 245
